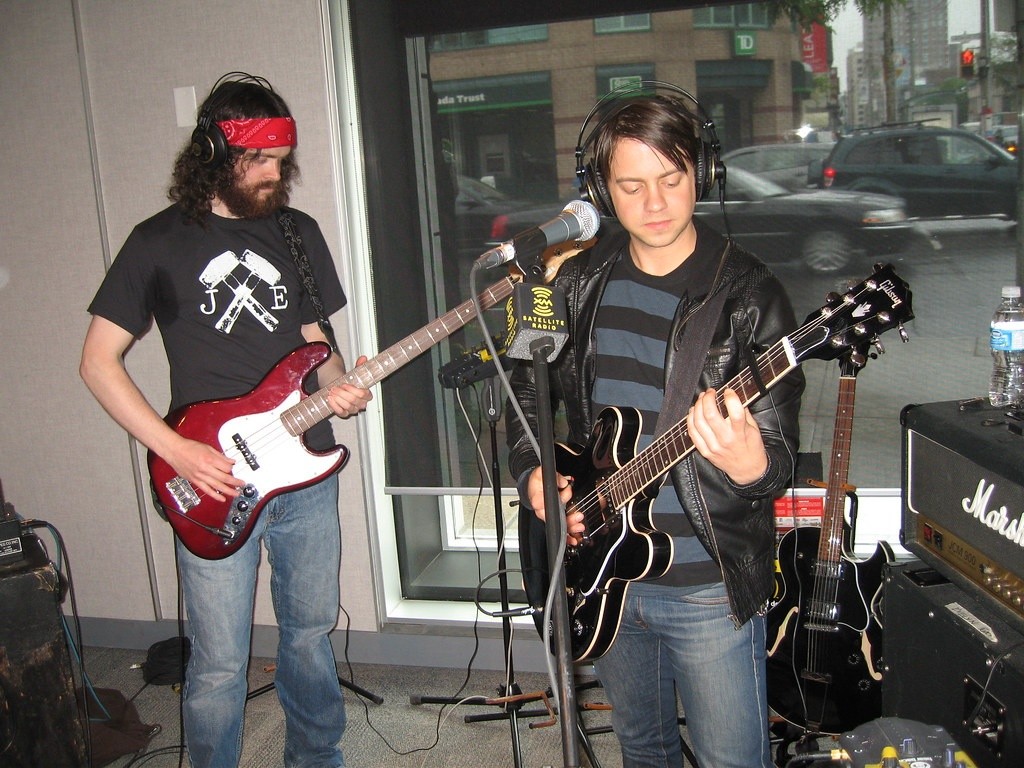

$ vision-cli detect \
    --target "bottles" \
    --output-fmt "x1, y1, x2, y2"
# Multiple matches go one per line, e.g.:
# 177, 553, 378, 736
990, 285, 1024, 406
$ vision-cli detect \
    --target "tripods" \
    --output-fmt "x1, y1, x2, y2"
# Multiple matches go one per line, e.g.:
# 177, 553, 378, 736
409, 376, 614, 768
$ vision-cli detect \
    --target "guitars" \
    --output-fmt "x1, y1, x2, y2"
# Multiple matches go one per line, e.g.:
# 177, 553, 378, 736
764, 340, 897, 737
147, 271, 524, 562
517, 259, 916, 668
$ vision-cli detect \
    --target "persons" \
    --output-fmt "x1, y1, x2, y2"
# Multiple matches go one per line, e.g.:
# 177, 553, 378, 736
78, 80, 372, 768
505, 94, 806, 768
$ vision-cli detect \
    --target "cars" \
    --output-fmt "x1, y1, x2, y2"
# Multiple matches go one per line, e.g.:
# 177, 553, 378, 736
959, 122, 1019, 156
455, 144, 940, 276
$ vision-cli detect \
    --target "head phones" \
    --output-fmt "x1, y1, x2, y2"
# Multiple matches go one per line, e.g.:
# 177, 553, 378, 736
576, 80, 728, 219
192, 70, 273, 168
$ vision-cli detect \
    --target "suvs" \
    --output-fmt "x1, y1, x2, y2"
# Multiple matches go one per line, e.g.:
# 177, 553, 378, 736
817, 118, 1019, 220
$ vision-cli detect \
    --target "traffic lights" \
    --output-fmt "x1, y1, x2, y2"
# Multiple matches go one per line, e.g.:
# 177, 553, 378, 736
960, 50, 974, 80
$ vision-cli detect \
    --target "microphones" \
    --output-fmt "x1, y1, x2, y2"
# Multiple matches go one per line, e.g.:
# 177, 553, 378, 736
473, 200, 601, 271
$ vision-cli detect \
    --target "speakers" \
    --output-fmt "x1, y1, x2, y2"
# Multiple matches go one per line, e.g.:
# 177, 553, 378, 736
880, 558, 1024, 768
0, 560, 89, 768
